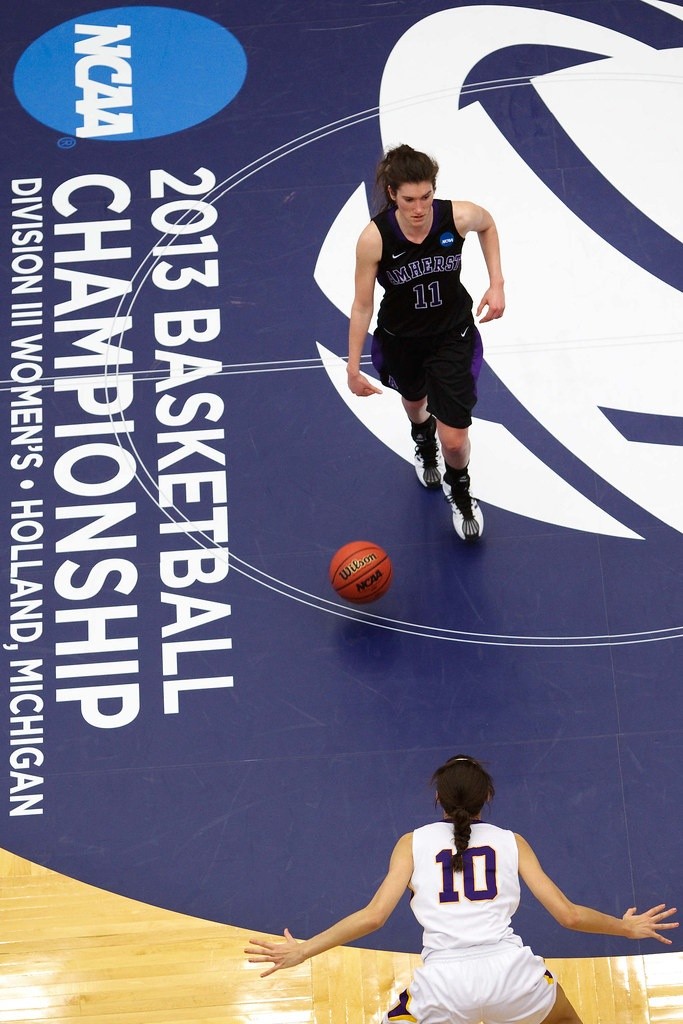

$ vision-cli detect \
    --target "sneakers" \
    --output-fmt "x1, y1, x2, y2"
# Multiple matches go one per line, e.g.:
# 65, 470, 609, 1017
407, 426, 447, 489
440, 473, 484, 540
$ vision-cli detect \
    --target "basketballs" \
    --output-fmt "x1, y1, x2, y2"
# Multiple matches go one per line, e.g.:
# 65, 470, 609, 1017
330, 540, 393, 603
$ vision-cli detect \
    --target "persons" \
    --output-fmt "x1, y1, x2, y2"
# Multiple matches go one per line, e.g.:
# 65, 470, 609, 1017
244, 754, 678, 1024
348, 143, 506, 541
